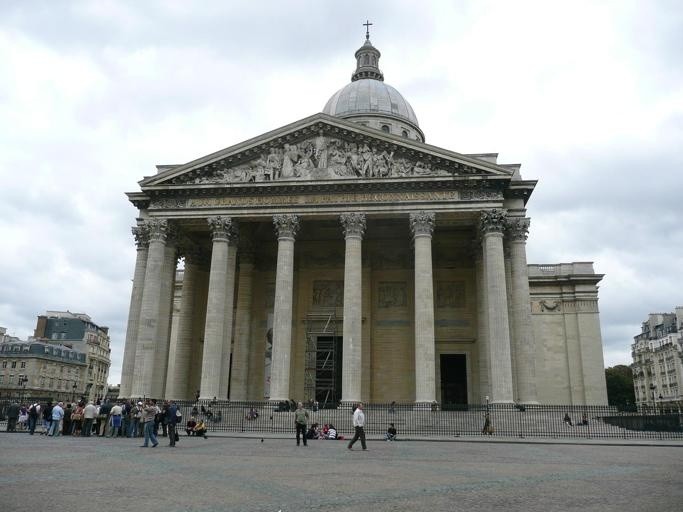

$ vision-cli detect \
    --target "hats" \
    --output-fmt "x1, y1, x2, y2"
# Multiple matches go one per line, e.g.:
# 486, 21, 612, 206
138, 402, 142, 406
47, 401, 53, 404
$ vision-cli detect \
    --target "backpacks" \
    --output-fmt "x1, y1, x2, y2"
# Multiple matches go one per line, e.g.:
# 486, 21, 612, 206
29, 404, 39, 416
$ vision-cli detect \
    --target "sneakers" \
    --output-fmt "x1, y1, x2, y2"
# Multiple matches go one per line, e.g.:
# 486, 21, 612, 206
166, 444, 175, 447
140, 442, 159, 447
347, 448, 367, 450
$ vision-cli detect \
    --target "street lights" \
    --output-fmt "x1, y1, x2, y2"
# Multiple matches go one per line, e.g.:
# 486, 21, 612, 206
20, 376, 28, 404
72, 383, 77, 402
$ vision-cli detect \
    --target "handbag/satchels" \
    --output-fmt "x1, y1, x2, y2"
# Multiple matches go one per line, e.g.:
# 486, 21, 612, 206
99, 414, 107, 420
176, 410, 182, 423
487, 425, 494, 432
47, 414, 52, 421
175, 430, 179, 441
71, 413, 81, 421
140, 415, 144, 423
154, 413, 163, 423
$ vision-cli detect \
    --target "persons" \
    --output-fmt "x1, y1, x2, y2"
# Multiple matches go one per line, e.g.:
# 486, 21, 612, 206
514, 398, 526, 412
198, 130, 432, 184
481, 412, 492, 436
162, 399, 177, 448
347, 402, 370, 452
562, 412, 572, 429
581, 413, 588, 425
384, 422, 397, 443
307, 422, 338, 440
429, 400, 437, 411
135, 398, 159, 448
277, 399, 318, 413
246, 407, 258, 420
193, 390, 199, 402
387, 401, 395, 413
8, 399, 168, 437
294, 401, 310, 446
184, 396, 223, 438
